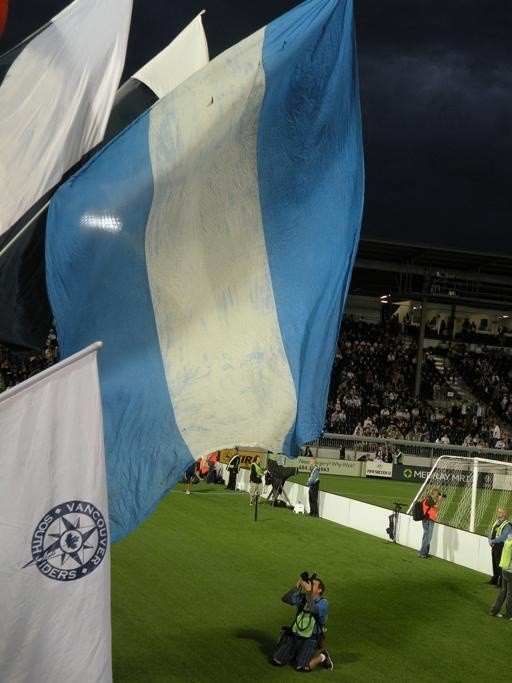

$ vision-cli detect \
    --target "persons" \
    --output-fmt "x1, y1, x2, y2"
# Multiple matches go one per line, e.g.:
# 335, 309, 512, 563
1, 340, 113, 683
488, 508, 512, 620
272, 572, 333, 672
326, 313, 511, 464
420, 488, 447, 558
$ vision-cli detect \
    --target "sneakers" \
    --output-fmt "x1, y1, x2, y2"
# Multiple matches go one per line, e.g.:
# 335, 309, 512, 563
320, 650, 334, 672
418, 554, 431, 559
495, 613, 504, 617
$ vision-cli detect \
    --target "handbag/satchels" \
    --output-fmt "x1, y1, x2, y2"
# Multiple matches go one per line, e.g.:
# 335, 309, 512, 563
292, 611, 316, 638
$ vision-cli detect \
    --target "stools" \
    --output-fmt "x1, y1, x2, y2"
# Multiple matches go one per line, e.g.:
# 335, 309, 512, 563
293, 504, 304, 514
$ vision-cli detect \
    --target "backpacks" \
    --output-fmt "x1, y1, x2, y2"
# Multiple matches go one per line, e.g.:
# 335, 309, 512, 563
412, 495, 434, 521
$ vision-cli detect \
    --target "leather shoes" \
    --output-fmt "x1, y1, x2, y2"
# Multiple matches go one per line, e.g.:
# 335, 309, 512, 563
485, 579, 497, 585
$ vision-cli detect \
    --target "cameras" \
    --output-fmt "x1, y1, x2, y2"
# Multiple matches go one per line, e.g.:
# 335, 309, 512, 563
301, 571, 316, 588
438, 493, 447, 498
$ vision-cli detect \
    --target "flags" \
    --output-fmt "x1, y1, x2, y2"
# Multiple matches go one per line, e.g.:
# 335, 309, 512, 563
0, 1, 365, 542
